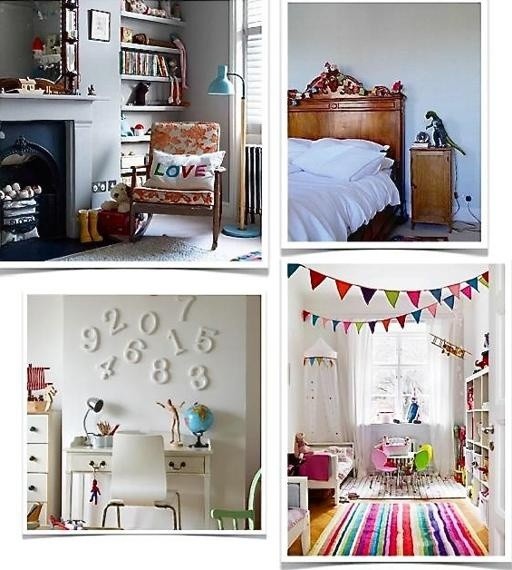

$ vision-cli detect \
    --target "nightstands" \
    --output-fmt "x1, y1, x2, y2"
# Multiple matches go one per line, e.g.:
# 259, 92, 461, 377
409, 148, 454, 233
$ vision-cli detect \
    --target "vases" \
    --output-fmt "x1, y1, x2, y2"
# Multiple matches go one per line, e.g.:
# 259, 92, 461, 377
379, 412, 394, 423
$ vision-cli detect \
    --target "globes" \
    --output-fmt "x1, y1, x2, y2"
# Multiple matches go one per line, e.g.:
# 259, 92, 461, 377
183, 402, 214, 448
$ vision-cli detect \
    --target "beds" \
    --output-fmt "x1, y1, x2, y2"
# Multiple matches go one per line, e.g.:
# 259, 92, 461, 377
288, 61, 407, 241
288, 442, 355, 507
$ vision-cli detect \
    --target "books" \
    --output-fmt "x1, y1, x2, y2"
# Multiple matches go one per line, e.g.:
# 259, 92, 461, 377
120, 51, 169, 77
411, 142, 429, 147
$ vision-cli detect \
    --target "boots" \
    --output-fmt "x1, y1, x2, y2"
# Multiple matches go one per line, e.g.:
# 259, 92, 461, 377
88, 209, 104, 242
78, 209, 92, 243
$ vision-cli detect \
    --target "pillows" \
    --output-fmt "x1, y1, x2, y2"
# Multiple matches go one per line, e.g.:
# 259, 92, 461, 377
288, 137, 395, 184
324, 446, 354, 462
142, 150, 225, 191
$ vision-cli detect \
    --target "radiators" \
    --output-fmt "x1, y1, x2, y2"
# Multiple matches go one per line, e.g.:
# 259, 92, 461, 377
244, 144, 262, 224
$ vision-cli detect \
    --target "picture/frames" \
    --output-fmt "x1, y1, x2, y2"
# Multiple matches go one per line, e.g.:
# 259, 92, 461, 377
88, 9, 111, 42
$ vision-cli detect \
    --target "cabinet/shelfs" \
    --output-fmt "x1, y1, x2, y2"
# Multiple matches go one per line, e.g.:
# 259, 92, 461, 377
121, 10, 184, 175
465, 367, 489, 521
27, 410, 62, 526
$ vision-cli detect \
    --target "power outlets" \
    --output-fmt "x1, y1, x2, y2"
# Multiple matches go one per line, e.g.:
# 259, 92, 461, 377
464, 195, 472, 204
454, 192, 460, 200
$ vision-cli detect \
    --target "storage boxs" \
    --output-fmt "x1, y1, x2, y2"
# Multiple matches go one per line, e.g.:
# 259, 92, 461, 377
121, 155, 144, 169
88, 208, 139, 235
465, 449, 489, 521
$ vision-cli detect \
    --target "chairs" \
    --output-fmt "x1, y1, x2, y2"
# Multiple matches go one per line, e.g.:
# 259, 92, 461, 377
103, 434, 181, 530
369, 442, 432, 496
129, 122, 224, 250
288, 477, 311, 556
211, 468, 261, 530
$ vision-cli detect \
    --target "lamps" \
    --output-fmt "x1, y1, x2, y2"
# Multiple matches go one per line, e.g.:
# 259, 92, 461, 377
82, 398, 104, 447
209, 66, 260, 238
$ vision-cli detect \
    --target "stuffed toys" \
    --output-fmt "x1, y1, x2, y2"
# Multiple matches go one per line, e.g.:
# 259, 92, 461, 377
295, 432, 313, 463
100, 183, 144, 221
126, 0, 182, 21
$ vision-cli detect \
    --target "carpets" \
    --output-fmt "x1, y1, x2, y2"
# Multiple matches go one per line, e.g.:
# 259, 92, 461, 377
41, 235, 234, 261
318, 503, 489, 556
392, 234, 448, 241
231, 251, 262, 261
341, 475, 467, 500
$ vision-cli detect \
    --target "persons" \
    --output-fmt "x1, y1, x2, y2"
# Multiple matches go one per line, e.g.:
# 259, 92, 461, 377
425, 109, 466, 156
169, 58, 181, 105
428, 331, 472, 362
169, 33, 189, 88
156, 399, 186, 446
90, 480, 101, 506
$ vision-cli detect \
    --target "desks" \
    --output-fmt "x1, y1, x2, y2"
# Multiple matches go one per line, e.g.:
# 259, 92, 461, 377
65, 444, 213, 530
